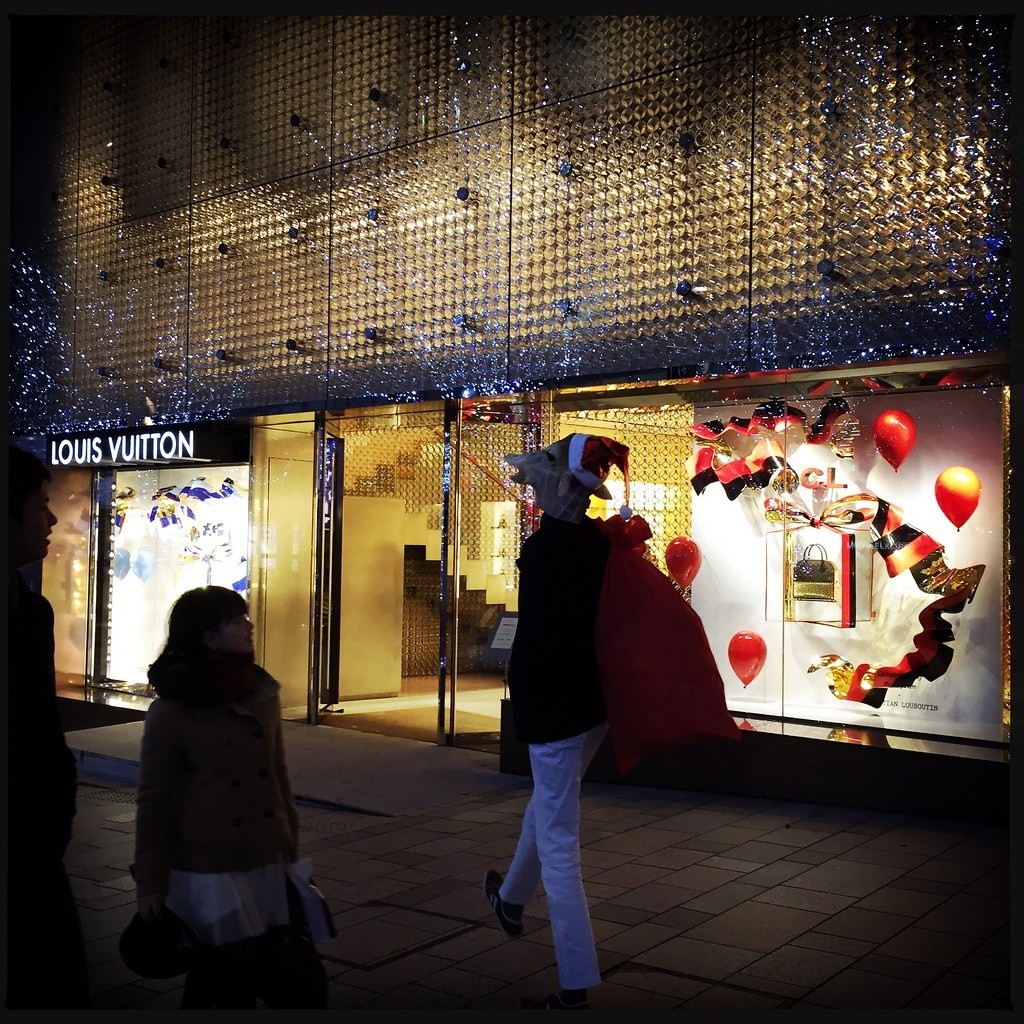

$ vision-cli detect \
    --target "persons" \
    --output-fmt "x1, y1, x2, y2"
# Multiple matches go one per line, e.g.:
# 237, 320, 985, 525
486, 433, 632, 1012
129, 586, 338, 1012
8, 445, 94, 1012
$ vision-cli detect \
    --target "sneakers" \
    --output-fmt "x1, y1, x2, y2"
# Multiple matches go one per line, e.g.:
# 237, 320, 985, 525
517, 993, 592, 1011
482, 868, 524, 938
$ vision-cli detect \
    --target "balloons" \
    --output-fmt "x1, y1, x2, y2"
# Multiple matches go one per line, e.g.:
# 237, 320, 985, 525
729, 631, 767, 690
872, 407, 917, 474
934, 466, 980, 531
665, 537, 702, 590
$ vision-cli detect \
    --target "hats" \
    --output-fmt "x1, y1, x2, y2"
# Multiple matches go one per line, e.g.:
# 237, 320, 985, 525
120, 904, 199, 978
570, 433, 630, 519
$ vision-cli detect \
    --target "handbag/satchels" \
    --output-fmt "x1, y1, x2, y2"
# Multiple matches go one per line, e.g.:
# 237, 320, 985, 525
597, 515, 742, 771
256, 872, 339, 1009
